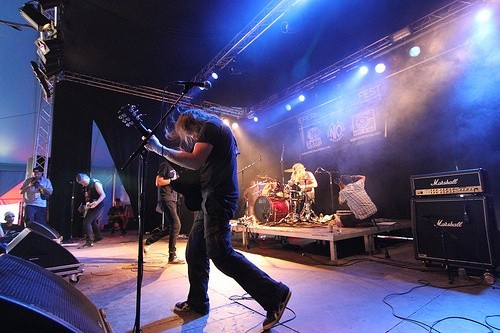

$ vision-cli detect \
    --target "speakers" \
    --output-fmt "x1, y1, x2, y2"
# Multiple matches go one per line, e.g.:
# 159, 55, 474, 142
27, 221, 62, 242
0, 252, 113, 333
6, 228, 85, 276
411, 195, 500, 275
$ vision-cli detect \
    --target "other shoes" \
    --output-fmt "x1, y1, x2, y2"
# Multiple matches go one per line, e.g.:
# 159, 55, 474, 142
93, 235, 103, 242
262, 288, 292, 330
142, 239, 148, 254
174, 301, 208, 315
168, 259, 184, 265
78, 243, 92, 249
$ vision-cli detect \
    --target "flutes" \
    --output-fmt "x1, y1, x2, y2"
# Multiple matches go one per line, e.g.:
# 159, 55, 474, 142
34, 179, 50, 199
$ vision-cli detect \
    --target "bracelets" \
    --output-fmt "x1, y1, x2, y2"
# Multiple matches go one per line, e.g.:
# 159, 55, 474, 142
161, 145, 164, 157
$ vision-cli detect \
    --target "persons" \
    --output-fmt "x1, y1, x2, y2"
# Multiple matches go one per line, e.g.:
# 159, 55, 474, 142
287, 162, 318, 214
337, 174, 378, 228
0, 211, 25, 253
19, 166, 54, 228
107, 197, 125, 239
141, 108, 293, 331
75, 172, 106, 248
142, 160, 186, 264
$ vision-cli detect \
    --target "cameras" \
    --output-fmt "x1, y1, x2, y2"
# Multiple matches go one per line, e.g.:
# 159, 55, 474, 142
32, 181, 40, 189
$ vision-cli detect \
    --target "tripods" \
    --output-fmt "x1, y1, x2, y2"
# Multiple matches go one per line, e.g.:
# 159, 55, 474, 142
280, 193, 317, 225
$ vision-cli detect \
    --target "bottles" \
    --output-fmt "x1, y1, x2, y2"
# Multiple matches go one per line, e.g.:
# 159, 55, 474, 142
335, 217, 341, 232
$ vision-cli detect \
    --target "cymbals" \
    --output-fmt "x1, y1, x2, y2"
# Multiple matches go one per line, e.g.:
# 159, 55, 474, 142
300, 179, 314, 180
261, 177, 271, 179
284, 168, 306, 173
276, 184, 292, 198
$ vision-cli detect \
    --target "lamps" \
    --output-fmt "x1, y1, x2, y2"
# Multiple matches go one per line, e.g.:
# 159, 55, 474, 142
30, 38, 66, 98
390, 25, 413, 44
281, 22, 298, 33
228, 65, 246, 75
211, 69, 224, 80
18, 3, 51, 32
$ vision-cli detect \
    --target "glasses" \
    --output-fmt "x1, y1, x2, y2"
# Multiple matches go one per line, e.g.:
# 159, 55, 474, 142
7, 216, 15, 218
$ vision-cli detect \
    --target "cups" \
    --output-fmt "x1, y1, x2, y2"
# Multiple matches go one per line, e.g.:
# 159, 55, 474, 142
328, 223, 333, 232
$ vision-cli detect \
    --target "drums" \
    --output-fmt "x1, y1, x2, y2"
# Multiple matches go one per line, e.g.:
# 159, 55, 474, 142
271, 180, 283, 192
257, 183, 268, 193
290, 190, 304, 201
254, 196, 289, 223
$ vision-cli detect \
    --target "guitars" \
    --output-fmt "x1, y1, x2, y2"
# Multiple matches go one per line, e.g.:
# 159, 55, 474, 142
117, 103, 204, 210
76, 201, 97, 217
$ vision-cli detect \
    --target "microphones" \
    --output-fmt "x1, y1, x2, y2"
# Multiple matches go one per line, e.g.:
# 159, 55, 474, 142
313, 168, 320, 174
462, 200, 469, 223
176, 81, 212, 90
